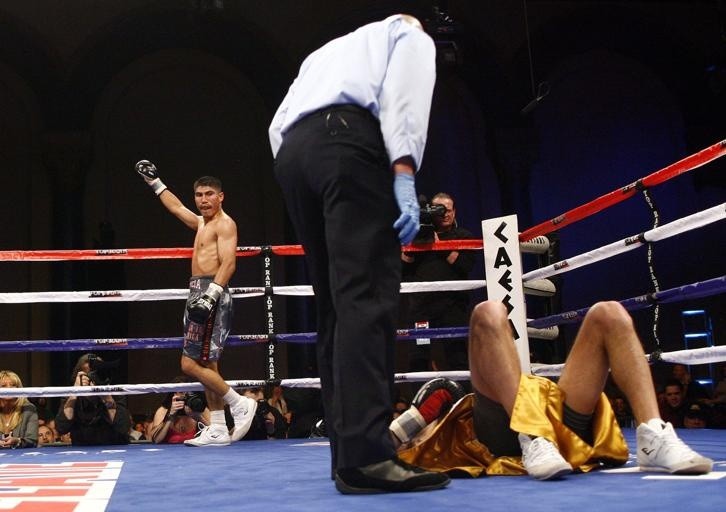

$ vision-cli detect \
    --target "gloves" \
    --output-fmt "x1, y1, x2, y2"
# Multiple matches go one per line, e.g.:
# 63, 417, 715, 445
391, 173, 422, 245
187, 282, 225, 324
135, 160, 167, 197
389, 377, 466, 443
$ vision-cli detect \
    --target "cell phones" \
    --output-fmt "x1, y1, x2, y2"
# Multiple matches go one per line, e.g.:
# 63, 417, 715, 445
2, 433, 11, 447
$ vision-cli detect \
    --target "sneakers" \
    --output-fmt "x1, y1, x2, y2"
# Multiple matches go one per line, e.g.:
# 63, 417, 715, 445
184, 425, 232, 447
231, 397, 259, 442
521, 436, 573, 482
635, 423, 713, 475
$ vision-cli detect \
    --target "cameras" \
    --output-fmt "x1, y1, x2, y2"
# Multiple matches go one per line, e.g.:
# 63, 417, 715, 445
176, 392, 206, 412
89, 354, 112, 385
257, 400, 270, 416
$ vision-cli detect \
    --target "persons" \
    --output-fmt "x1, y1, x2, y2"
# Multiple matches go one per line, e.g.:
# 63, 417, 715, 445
606, 364, 726, 429
228, 384, 327, 440
134, 157, 260, 448
397, 190, 478, 398
388, 301, 715, 481
1, 354, 209, 448
393, 402, 406, 419
264, 3, 454, 496
